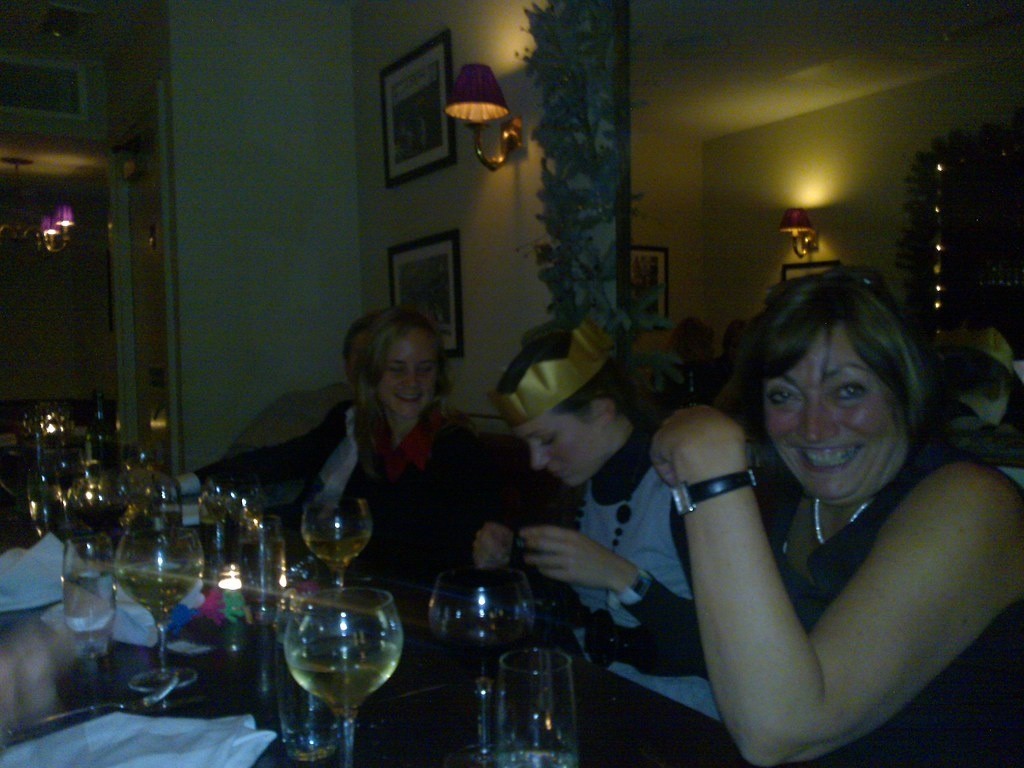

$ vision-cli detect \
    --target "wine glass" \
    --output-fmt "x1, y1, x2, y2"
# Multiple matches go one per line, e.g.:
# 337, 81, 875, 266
285, 588, 404, 768
112, 525, 204, 694
428, 565, 535, 768
300, 495, 373, 589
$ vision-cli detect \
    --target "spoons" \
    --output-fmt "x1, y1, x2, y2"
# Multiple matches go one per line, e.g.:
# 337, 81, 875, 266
8, 674, 177, 732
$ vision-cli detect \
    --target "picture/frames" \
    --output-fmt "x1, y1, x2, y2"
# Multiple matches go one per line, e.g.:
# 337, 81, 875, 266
782, 260, 839, 281
380, 28, 458, 188
388, 229, 463, 358
630, 244, 669, 330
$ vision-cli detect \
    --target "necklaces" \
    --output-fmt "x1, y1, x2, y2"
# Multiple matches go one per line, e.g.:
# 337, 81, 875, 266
570, 465, 632, 619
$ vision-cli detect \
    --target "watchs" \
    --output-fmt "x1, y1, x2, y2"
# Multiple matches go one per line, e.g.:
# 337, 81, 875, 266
671, 467, 761, 515
618, 567, 652, 606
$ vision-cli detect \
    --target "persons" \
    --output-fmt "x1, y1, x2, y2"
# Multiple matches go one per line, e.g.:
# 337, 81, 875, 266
650, 264, 1024, 768
173, 307, 506, 586
471, 316, 722, 721
921, 323, 1024, 494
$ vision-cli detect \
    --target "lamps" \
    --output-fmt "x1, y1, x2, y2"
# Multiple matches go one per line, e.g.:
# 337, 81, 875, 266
443, 63, 522, 171
0, 157, 76, 253
779, 208, 818, 257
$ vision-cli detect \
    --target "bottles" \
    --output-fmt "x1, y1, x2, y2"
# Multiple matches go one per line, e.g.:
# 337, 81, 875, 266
84, 387, 118, 471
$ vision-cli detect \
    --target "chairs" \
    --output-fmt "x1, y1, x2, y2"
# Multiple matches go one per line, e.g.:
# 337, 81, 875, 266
479, 431, 578, 561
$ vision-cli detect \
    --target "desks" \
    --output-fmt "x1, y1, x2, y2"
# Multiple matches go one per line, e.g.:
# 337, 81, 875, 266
0, 526, 849, 768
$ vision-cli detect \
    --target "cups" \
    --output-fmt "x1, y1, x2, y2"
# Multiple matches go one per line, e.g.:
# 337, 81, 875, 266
23, 400, 290, 694
501, 647, 579, 768
61, 530, 116, 633
272, 638, 337, 762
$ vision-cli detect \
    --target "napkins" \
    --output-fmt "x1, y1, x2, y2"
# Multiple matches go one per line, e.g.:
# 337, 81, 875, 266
0, 532, 101, 613
0, 712, 278, 766
39, 576, 158, 648
